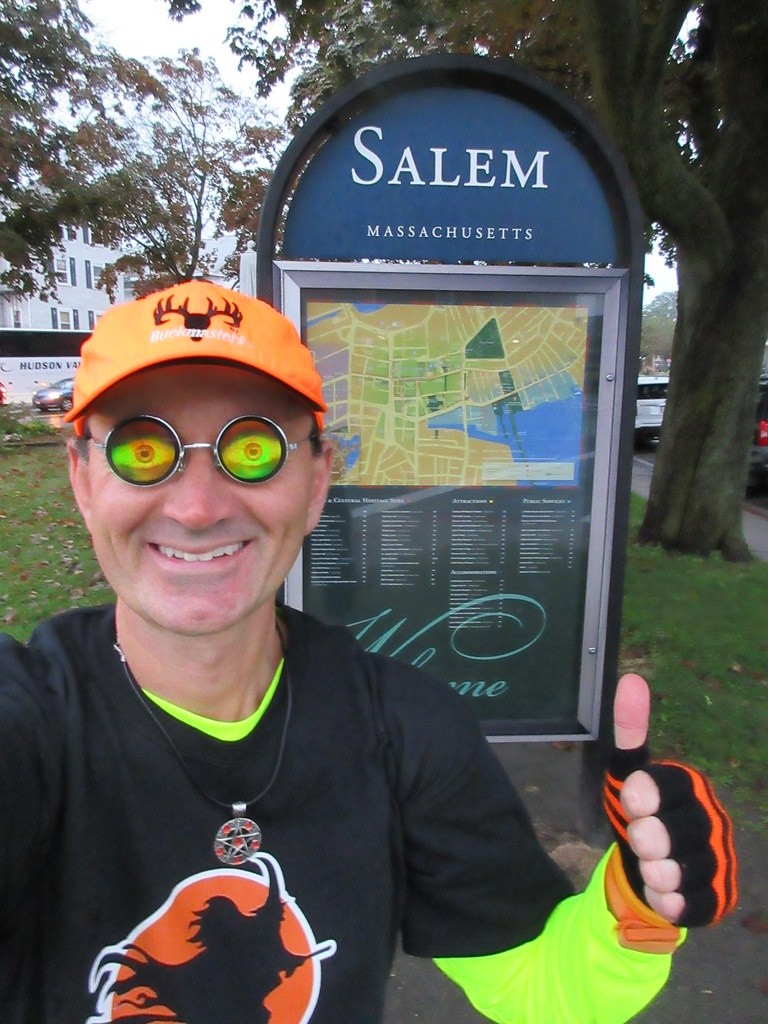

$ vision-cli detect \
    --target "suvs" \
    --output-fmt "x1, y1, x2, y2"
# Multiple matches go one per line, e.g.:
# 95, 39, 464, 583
635, 376, 670, 448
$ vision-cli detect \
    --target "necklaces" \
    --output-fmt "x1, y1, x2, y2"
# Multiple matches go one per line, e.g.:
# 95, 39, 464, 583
114, 622, 293, 865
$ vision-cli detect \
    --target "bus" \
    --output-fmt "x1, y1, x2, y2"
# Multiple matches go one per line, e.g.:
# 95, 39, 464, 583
1, 328, 94, 395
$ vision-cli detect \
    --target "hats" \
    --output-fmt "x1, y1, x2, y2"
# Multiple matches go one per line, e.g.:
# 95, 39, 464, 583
63, 277, 326, 434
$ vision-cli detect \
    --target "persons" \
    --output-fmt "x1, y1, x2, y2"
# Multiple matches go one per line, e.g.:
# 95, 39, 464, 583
0, 280, 736, 1023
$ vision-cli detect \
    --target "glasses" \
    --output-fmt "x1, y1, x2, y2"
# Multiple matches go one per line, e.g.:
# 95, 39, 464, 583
88, 411, 314, 485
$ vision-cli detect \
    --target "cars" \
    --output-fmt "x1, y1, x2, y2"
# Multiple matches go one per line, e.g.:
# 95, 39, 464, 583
751, 381, 768, 472
32, 378, 74, 412
